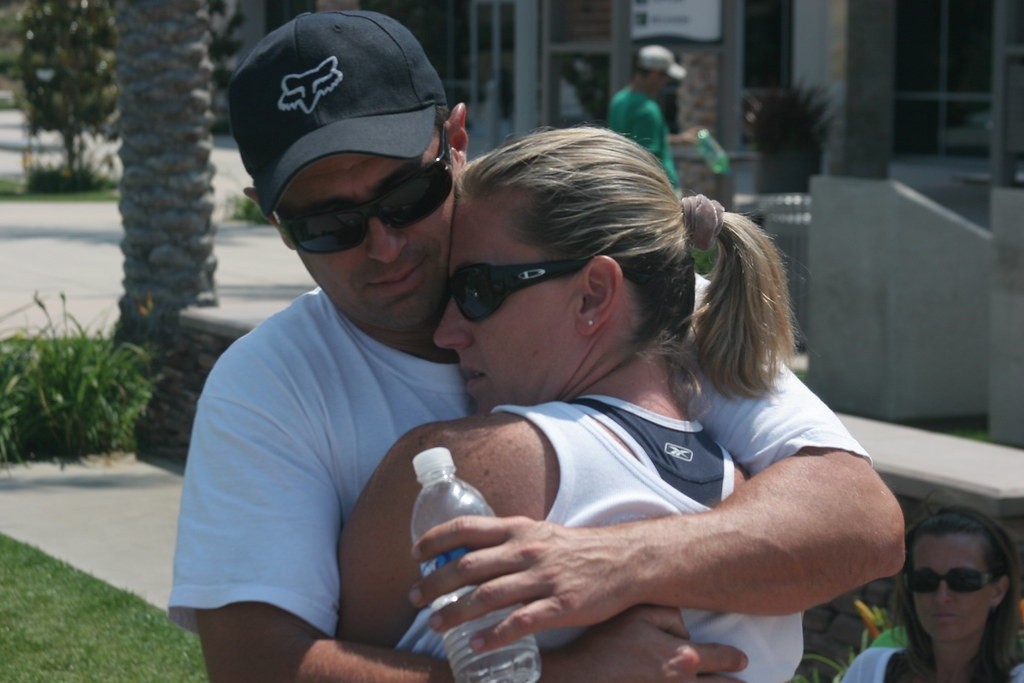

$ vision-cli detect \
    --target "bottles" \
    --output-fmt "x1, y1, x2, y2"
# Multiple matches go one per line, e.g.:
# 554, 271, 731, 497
692, 128, 733, 177
410, 446, 543, 683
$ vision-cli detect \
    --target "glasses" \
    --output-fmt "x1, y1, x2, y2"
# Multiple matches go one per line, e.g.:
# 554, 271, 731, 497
270, 122, 451, 254
446, 259, 590, 321
908, 565, 998, 593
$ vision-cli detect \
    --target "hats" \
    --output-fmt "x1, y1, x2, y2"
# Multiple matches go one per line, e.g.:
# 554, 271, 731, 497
638, 44, 687, 80
226, 10, 448, 221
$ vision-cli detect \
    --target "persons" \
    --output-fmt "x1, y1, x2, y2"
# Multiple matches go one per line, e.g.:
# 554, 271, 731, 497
837, 502, 1024, 683
173, 10, 906, 683
609, 46, 705, 196
